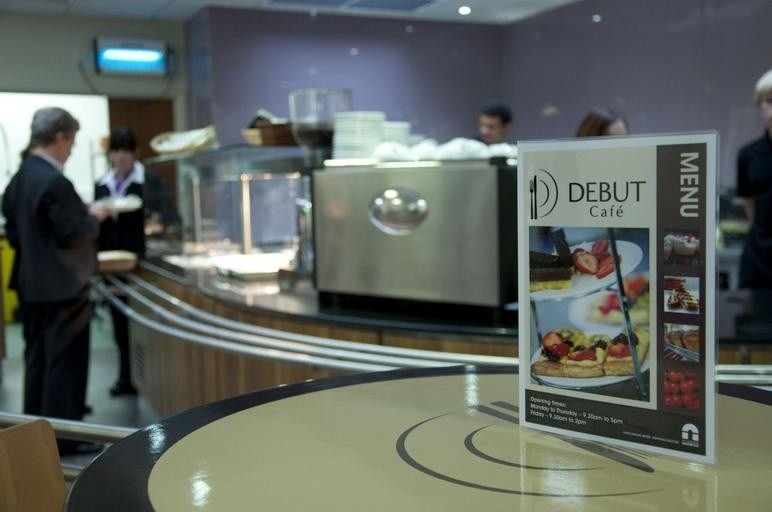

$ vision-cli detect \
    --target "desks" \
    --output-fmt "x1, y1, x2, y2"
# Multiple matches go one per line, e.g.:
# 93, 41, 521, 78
60, 362, 772, 512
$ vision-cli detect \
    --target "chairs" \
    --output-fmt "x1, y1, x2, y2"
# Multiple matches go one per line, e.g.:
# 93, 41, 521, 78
0, 422, 67, 512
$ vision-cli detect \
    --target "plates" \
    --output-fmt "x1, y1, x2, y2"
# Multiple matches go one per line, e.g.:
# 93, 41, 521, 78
530, 240, 650, 389
332, 111, 425, 163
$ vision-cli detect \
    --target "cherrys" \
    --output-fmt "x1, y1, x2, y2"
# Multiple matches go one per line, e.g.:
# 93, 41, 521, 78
663, 371, 701, 412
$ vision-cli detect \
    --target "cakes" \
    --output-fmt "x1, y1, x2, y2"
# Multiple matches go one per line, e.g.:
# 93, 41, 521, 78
531, 276, 649, 378
529, 250, 573, 292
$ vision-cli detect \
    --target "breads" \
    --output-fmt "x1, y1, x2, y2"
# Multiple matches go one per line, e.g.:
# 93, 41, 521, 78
664, 329, 700, 353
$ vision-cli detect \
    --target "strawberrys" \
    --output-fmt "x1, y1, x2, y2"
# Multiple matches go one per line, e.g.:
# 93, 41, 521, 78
573, 239, 622, 279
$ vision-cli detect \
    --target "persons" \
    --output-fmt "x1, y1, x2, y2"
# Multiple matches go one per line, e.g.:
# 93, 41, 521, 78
574, 107, 631, 137
735, 70, 772, 346
4, 108, 111, 456
94, 129, 172, 396
474, 105, 512, 145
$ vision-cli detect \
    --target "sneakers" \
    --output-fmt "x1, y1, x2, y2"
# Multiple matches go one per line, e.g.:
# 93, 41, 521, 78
58, 438, 103, 458
